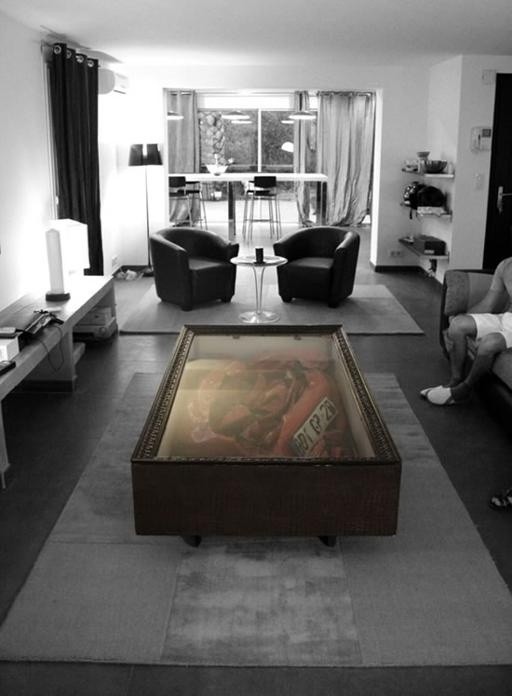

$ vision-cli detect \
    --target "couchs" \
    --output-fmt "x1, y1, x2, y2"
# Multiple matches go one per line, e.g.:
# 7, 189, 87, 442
439, 269, 511, 435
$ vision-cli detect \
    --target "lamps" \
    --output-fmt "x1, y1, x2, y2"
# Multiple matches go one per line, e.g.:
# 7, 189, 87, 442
129, 144, 162, 277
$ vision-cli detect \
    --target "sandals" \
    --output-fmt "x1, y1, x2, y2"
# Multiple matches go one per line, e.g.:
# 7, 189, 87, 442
488, 487, 512, 512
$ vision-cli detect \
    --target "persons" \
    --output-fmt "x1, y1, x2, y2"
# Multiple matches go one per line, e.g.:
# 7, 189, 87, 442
418, 254, 511, 405
489, 482, 511, 514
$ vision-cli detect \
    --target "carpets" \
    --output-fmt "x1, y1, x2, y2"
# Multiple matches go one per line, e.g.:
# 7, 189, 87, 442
121, 284, 425, 335
0, 372, 512, 666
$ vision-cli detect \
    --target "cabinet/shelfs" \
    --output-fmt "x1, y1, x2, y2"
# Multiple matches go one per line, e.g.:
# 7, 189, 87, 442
398, 168, 454, 260
1, 276, 118, 475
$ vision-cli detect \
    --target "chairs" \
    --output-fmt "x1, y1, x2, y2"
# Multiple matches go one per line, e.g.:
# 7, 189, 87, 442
273, 226, 360, 308
169, 177, 208, 229
150, 227, 239, 311
243, 176, 282, 247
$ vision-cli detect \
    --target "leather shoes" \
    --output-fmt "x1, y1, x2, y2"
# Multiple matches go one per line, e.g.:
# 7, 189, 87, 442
420, 385, 471, 406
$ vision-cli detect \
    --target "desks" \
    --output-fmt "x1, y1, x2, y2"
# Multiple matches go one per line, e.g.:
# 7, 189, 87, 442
230, 256, 288, 324
169, 173, 330, 226
130, 325, 401, 546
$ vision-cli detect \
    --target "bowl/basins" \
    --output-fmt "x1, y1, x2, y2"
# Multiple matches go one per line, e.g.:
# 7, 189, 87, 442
206, 164, 228, 176
419, 160, 447, 174
